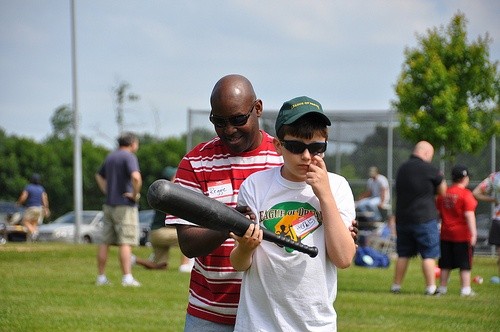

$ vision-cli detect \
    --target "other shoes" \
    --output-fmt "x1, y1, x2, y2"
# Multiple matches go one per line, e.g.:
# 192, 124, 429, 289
94, 280, 113, 286
461, 290, 479, 297
425, 290, 441, 296
121, 279, 140, 286
390, 289, 400, 294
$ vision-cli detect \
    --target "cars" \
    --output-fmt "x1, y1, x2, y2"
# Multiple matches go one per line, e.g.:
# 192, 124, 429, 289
36, 209, 105, 245
1, 201, 30, 241
138, 209, 158, 245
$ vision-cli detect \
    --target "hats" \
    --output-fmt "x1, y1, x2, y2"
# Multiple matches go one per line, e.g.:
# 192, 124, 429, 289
275, 96, 331, 132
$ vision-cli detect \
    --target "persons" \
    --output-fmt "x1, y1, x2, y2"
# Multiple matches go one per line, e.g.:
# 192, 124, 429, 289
15, 174, 50, 243
388, 141, 448, 297
93, 133, 144, 287
439, 166, 478, 296
473, 172, 500, 283
367, 164, 391, 221
230, 95, 356, 332
163, 74, 361, 332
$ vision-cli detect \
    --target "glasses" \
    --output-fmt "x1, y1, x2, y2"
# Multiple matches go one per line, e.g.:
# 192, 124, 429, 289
278, 139, 327, 154
209, 101, 256, 128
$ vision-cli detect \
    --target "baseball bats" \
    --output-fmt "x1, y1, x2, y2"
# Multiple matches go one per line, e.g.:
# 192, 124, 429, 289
146, 179, 318, 258
